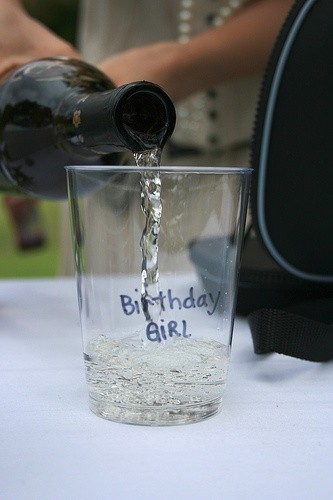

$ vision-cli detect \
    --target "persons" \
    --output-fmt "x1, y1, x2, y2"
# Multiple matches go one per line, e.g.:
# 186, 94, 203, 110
0, 1, 293, 271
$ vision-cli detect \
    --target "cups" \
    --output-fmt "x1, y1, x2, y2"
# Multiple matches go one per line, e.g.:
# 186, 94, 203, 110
64, 164, 253, 427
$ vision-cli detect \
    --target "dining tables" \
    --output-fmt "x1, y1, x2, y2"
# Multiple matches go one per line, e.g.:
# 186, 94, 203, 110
0, 277, 332, 498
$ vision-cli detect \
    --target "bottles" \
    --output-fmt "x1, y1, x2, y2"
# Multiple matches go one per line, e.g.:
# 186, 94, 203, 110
0, 56, 178, 202
